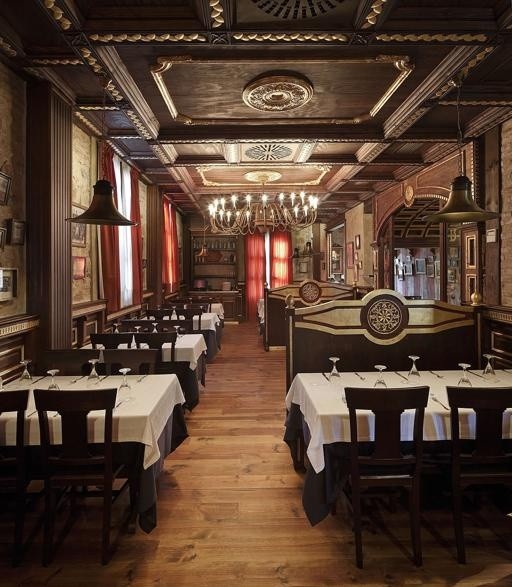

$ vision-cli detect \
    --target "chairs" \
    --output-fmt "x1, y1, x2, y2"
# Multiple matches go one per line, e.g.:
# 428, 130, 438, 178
330, 386, 430, 569
432, 385, 512, 565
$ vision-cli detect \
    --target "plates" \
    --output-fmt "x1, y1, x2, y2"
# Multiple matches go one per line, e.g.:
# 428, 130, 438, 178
223, 282, 231, 290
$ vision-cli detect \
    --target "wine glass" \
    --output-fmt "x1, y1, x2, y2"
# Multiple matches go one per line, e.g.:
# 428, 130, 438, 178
112, 322, 181, 334
456, 354, 495, 386
117, 368, 132, 402
46, 369, 61, 390
373, 365, 387, 388
329, 357, 340, 382
407, 355, 420, 381
19, 360, 31, 385
87, 358, 100, 388
171, 305, 177, 319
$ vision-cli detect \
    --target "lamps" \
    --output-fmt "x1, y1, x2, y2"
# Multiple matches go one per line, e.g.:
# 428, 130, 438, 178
286, 247, 303, 259
208, 175, 319, 235
420, 86, 503, 224
65, 88, 138, 227
301, 238, 322, 256
195, 215, 212, 258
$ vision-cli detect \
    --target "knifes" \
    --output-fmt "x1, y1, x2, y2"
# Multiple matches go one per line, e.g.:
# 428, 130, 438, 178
394, 371, 407, 380
96, 374, 109, 384
32, 375, 46, 384
322, 372, 330, 381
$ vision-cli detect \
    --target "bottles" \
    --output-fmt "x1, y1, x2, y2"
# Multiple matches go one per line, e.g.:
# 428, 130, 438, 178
194, 238, 236, 249
194, 252, 208, 264
223, 253, 236, 262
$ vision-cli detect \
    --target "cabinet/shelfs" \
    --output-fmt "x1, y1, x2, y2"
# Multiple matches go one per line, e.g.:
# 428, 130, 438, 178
191, 235, 240, 290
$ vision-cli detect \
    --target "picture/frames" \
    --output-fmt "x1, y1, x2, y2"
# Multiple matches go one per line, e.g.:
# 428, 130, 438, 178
394, 248, 459, 283
72, 256, 86, 280
0, 267, 19, 302
345, 235, 362, 282
0, 226, 7, 256
9, 217, 24, 246
71, 202, 89, 248
0, 170, 14, 206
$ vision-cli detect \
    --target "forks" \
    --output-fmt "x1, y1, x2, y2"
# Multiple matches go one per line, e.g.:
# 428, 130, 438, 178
430, 393, 450, 411
